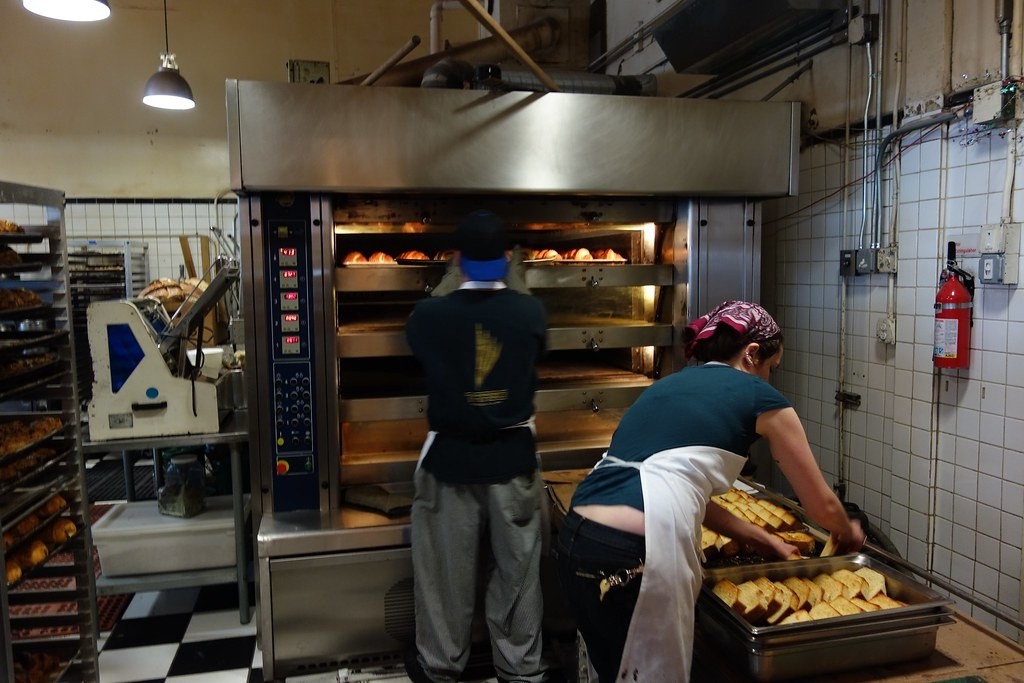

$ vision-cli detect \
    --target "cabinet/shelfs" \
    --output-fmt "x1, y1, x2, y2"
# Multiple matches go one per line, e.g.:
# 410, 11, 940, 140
0, 180, 150, 683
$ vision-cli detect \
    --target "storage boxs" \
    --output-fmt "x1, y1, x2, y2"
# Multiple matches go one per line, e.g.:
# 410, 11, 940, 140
92, 502, 239, 580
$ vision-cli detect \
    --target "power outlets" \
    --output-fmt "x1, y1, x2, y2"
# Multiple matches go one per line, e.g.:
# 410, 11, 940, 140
876, 318, 896, 346
856, 249, 877, 274
877, 247, 898, 273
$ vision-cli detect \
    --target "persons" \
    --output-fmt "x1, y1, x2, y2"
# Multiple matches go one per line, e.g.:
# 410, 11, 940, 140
548, 301, 865, 683
405, 231, 548, 683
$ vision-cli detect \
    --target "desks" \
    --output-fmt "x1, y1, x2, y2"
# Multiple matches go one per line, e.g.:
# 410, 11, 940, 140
79, 407, 256, 626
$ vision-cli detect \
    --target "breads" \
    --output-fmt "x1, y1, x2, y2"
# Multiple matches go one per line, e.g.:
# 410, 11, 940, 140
0, 217, 76, 582
698, 487, 908, 628
344, 246, 623, 263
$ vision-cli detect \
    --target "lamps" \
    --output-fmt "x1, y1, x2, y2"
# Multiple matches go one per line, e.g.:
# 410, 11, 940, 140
22, 0, 111, 22
142, 0, 197, 110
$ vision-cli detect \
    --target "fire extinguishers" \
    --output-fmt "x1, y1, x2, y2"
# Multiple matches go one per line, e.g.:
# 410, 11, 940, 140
933, 239, 976, 369
